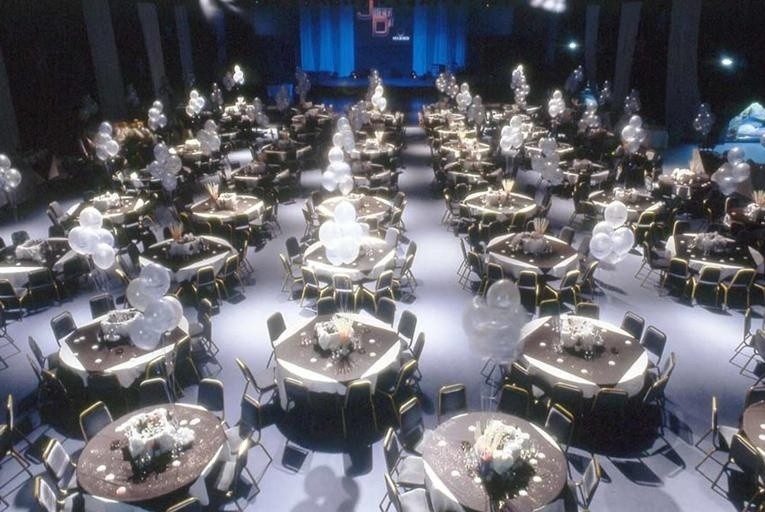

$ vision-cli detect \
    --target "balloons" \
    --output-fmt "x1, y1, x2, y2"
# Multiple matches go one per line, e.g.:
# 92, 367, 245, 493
467, 95, 485, 124
148, 99, 170, 132
618, 85, 649, 154
462, 278, 531, 364
434, 73, 460, 100
0, 154, 22, 194
500, 61, 615, 187
146, 145, 183, 191
713, 145, 753, 197
588, 200, 634, 266
692, 101, 718, 137
456, 83, 473, 110
95, 121, 120, 161
125, 265, 185, 349
66, 206, 115, 270
318, 202, 366, 262
321, 70, 387, 195
185, 62, 314, 158
